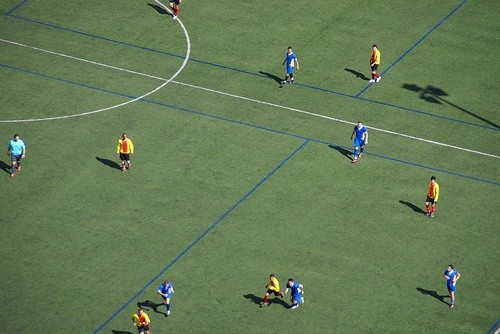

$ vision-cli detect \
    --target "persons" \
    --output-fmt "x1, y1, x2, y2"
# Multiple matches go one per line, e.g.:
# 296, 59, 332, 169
260, 274, 284, 308
283, 46, 299, 83
425, 176, 440, 217
7, 134, 25, 177
285, 278, 305, 310
170, 0, 182, 19
368, 44, 382, 82
117, 133, 134, 173
443, 265, 461, 308
158, 280, 175, 315
131, 309, 151, 334
350, 121, 368, 163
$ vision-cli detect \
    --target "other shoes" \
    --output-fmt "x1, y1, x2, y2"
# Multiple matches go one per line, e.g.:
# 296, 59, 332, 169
376, 76, 381, 82
430, 213, 434, 217
10, 173, 15, 177
359, 154, 362, 158
281, 292, 284, 297
291, 303, 299, 308
281, 80, 288, 83
18, 167, 21, 171
450, 304, 454, 308
260, 303, 267, 307
127, 164, 131, 169
368, 79, 375, 83
289, 79, 294, 83
166, 310, 170, 316
300, 297, 304, 304
173, 14, 177, 19
426, 212, 430, 216
351, 159, 357, 162
121, 169, 126, 171
